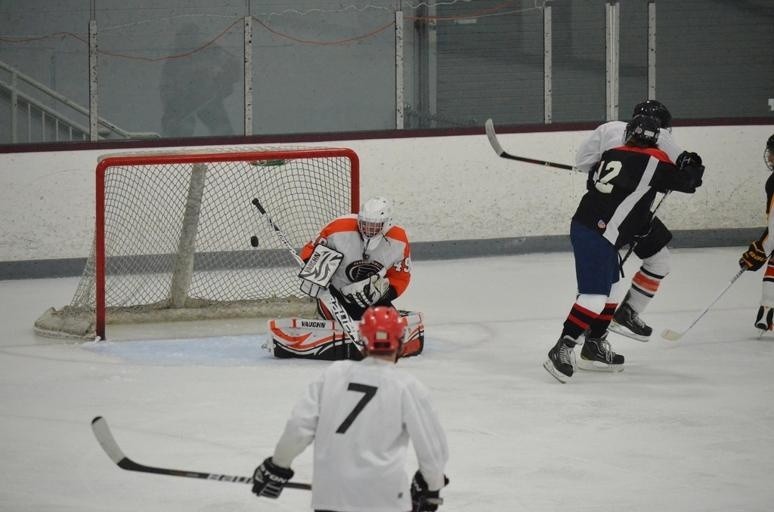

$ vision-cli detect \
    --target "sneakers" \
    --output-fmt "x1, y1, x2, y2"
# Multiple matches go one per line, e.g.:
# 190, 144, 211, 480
547, 336, 579, 377
754, 305, 770, 331
580, 328, 625, 365
613, 289, 653, 336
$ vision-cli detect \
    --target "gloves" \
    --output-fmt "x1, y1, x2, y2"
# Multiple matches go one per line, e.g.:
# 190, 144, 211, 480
409, 468, 450, 512
250, 456, 295, 499
676, 150, 702, 175
738, 239, 769, 272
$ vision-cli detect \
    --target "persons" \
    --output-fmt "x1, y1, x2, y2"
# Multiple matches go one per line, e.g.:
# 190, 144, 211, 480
572, 98, 701, 339
546, 113, 706, 376
157, 25, 239, 136
250, 303, 449, 512
736, 134, 774, 332
259, 195, 425, 362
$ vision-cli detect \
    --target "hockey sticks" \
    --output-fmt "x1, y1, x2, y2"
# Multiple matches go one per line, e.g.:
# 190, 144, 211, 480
483, 118, 579, 171
659, 265, 749, 341
91, 416, 310, 490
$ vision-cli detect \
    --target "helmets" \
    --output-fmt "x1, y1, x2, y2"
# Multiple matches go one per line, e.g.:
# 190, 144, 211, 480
359, 306, 404, 354
762, 134, 774, 171
620, 115, 661, 148
357, 196, 392, 239
632, 100, 672, 131
